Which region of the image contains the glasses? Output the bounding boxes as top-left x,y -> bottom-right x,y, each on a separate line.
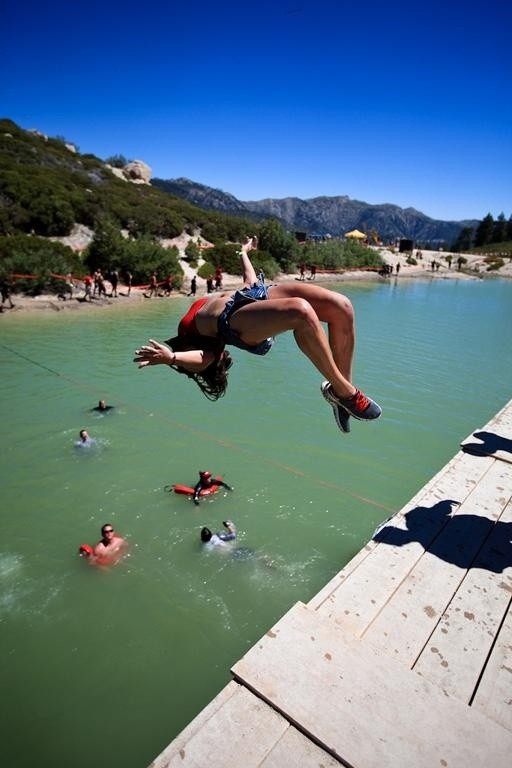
102,530 -> 114,533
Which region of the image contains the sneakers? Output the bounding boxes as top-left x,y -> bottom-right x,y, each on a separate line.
319,380 -> 382,433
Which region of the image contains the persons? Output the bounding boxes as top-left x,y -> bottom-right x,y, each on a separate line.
432,259 -> 435,273
310,261 -> 318,280
299,262 -> 308,280
55,268 -> 183,303
74,430 -> 97,450
0,277 -> 15,313
133,234 -> 383,434
193,471 -> 232,506
88,400 -> 113,416
416,252 -> 418,260
383,261 -> 401,276
198,521 -> 237,553
92,523 -> 126,559
206,263 -> 224,293
436,265 -> 439,271
259,267 -> 265,283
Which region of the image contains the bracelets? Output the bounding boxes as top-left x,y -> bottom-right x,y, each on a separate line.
170,354 -> 175,366
